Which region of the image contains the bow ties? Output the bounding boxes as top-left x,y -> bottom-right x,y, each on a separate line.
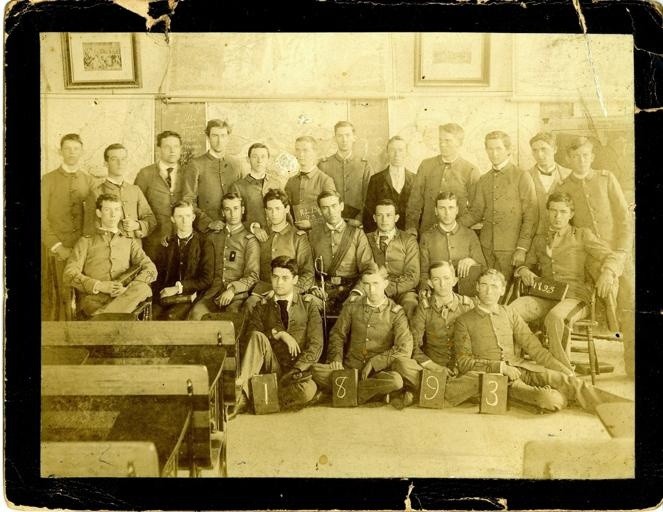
179,238 -> 188,242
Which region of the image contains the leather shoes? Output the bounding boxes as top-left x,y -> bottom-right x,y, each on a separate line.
305,390 -> 331,407
226,393 -> 248,419
402,388 -> 419,407
384,392 -> 404,410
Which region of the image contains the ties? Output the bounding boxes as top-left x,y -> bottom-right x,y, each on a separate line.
548,232 -> 558,249
166,168 -> 173,188
277,300 -> 289,329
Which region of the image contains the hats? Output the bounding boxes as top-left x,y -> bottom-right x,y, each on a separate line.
281,370 -> 313,386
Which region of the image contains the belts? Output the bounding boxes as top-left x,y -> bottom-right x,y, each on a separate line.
324,276 -> 349,286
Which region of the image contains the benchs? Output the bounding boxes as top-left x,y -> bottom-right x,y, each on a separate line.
41,320 -> 233,479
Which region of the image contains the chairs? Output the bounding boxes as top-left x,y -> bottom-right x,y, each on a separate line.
565,284 -> 599,381
51,255 -> 152,320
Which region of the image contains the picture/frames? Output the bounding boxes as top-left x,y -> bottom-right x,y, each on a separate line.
60,34 -> 144,91
413,31 -> 495,89
